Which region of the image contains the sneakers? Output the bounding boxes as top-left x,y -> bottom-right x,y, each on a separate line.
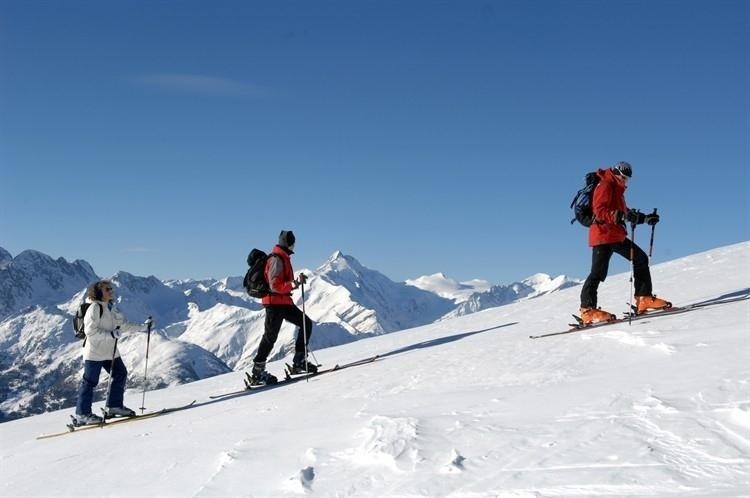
292,351 -> 317,372
579,307 -> 611,324
251,362 -> 277,385
103,406 -> 134,417
634,295 -> 667,313
75,412 -> 105,425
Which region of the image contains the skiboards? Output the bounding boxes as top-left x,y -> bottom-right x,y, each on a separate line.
36,399 -> 199,441
530,289 -> 750,338
209,356 -> 376,398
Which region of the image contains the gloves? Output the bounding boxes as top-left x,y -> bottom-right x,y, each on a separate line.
640,212 -> 658,225
293,273 -> 307,288
110,328 -> 122,339
615,209 -> 646,225
145,319 -> 156,331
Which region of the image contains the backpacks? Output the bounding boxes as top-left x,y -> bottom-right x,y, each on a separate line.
570,172 -> 601,228
238,247 -> 282,298
72,301 -> 103,345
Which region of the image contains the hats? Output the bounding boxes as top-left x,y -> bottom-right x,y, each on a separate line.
276,228 -> 294,249
614,161 -> 634,179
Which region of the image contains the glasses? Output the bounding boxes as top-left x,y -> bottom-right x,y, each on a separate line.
105,287 -> 113,292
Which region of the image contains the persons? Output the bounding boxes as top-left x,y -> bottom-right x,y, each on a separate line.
571,161 -> 672,323
243,230 -> 319,380
73,281 -> 136,423
